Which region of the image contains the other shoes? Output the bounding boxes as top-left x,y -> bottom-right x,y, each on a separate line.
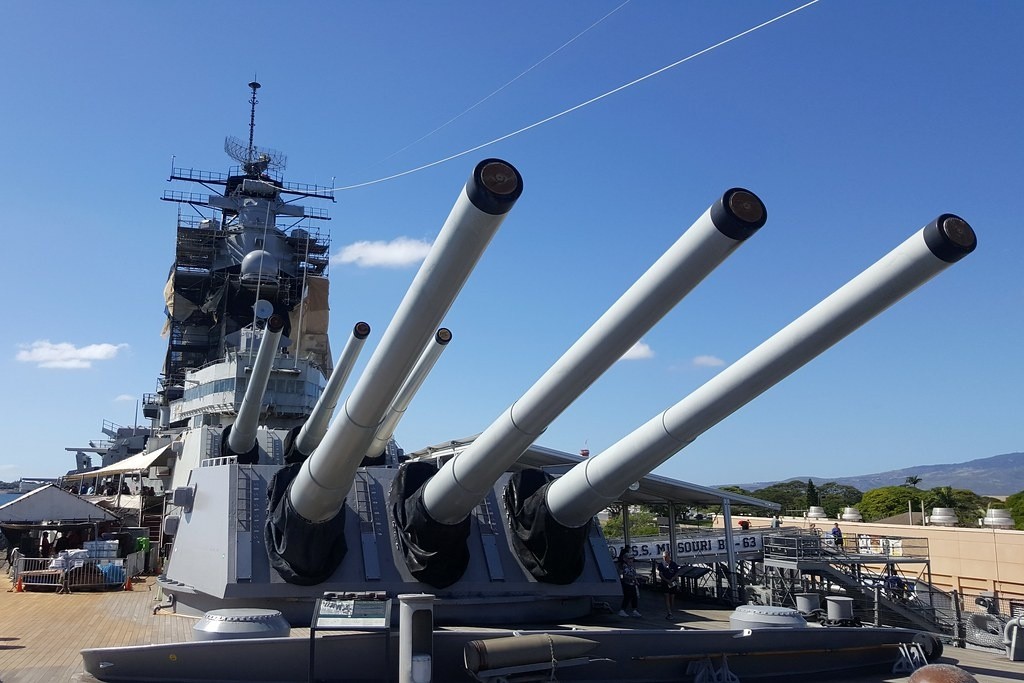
666,613 -> 675,620
618,610 -> 629,617
632,611 -> 642,617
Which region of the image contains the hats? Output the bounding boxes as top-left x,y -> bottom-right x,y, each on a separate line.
43,532 -> 49,535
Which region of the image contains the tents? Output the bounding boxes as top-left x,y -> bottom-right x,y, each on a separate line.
61,431 -> 183,526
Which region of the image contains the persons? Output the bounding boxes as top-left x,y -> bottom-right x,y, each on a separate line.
42,532 -> 54,559
55,531 -> 81,554
64,482 -> 131,495
